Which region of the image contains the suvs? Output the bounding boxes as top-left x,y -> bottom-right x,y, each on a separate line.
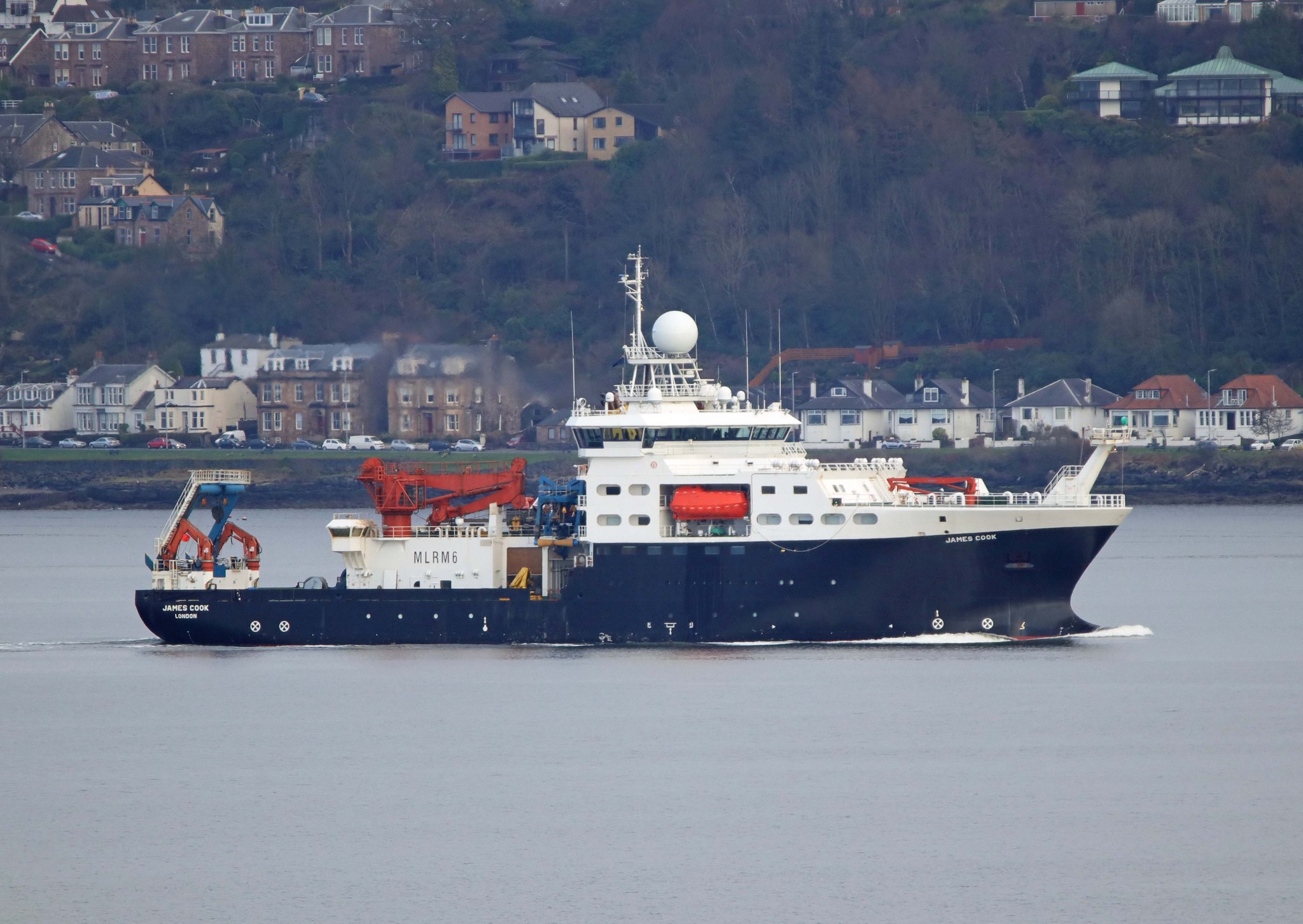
218,438 -> 270,450
390,440 -> 417,451
1280,439 -> 1302,451
429,439 -> 486,452
322,439 -> 350,451
881,438 -> 911,449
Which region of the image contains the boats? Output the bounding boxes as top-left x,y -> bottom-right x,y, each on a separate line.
133,244 -> 1135,645
670,486 -> 747,519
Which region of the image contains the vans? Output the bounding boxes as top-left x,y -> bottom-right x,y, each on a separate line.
349,435 -> 385,450
215,430 -> 246,446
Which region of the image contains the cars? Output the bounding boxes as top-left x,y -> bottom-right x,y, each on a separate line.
12,437 -> 52,448
290,440 -> 321,450
506,435 -> 522,447
89,437 -> 120,449
147,438 -> 186,450
1249,439 -> 1275,450
58,438 -> 86,449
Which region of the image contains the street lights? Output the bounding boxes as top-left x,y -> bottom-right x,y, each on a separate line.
791,372 -> 800,442
993,369 -> 1001,448
166,371 -> 173,449
20,370 -> 30,449
1209,369 -> 1217,441
